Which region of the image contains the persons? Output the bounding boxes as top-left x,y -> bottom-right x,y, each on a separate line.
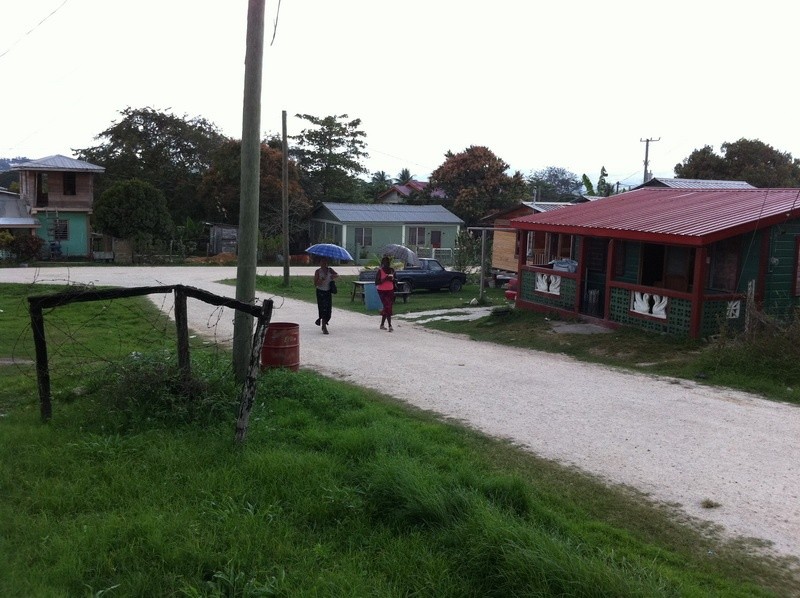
374,256 -> 398,332
314,258 -> 338,335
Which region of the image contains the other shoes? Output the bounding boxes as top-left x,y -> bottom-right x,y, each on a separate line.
315,319 -> 320,325
322,329 -> 329,334
388,326 -> 394,332
380,325 -> 387,330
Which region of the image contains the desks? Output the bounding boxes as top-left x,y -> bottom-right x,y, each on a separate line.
352,279 -> 412,306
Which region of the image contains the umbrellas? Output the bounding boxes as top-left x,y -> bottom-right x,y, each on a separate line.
380,244 -> 420,279
304,243 -> 354,280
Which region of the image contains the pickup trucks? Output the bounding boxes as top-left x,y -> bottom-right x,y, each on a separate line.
360,258 -> 467,293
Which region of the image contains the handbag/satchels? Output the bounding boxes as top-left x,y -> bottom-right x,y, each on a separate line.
329,280 -> 337,294
390,268 -> 399,290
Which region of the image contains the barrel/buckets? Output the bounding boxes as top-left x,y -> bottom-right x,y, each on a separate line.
261,323 -> 300,371
